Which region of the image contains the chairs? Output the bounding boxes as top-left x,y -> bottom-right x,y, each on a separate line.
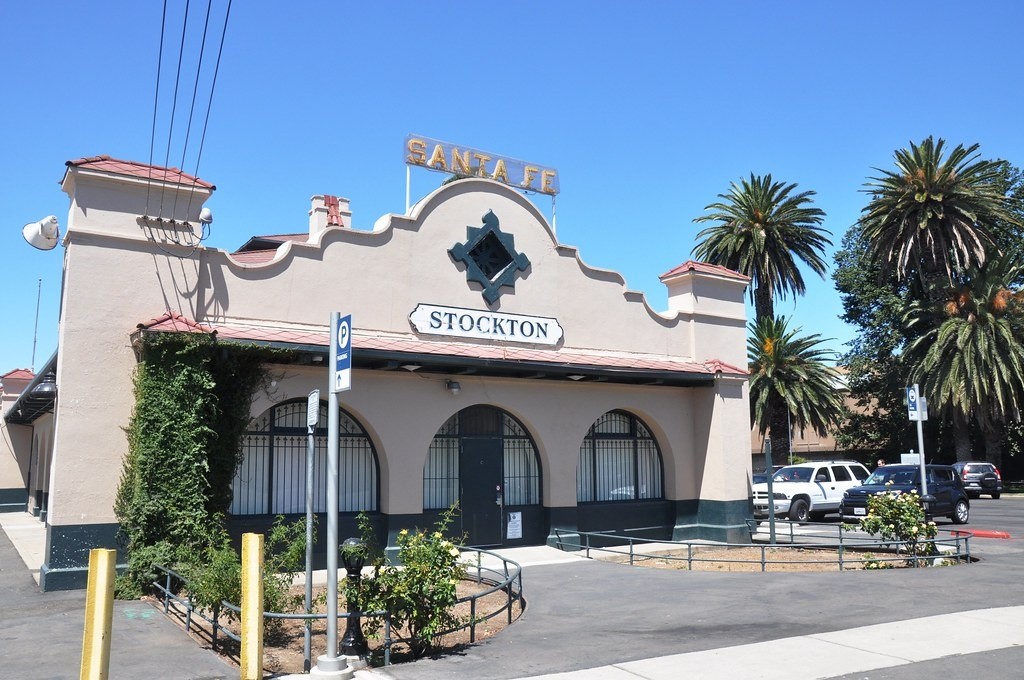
884,472 -> 901,485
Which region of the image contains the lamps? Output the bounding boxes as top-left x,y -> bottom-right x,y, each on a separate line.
310,354 -> 325,362
30,366 -> 60,397
23,215 -> 69,251
400,360 -> 423,372
446,381 -> 462,396
566,374 -> 590,382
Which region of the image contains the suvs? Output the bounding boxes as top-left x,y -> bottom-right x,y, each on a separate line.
753,461 -> 871,525
839,464 -> 970,524
951,462 -> 1002,500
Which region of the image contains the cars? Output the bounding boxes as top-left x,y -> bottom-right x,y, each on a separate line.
753,465 -> 787,484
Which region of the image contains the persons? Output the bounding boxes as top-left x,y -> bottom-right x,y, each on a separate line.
794,471 -> 800,478
872,459 -> 886,484
